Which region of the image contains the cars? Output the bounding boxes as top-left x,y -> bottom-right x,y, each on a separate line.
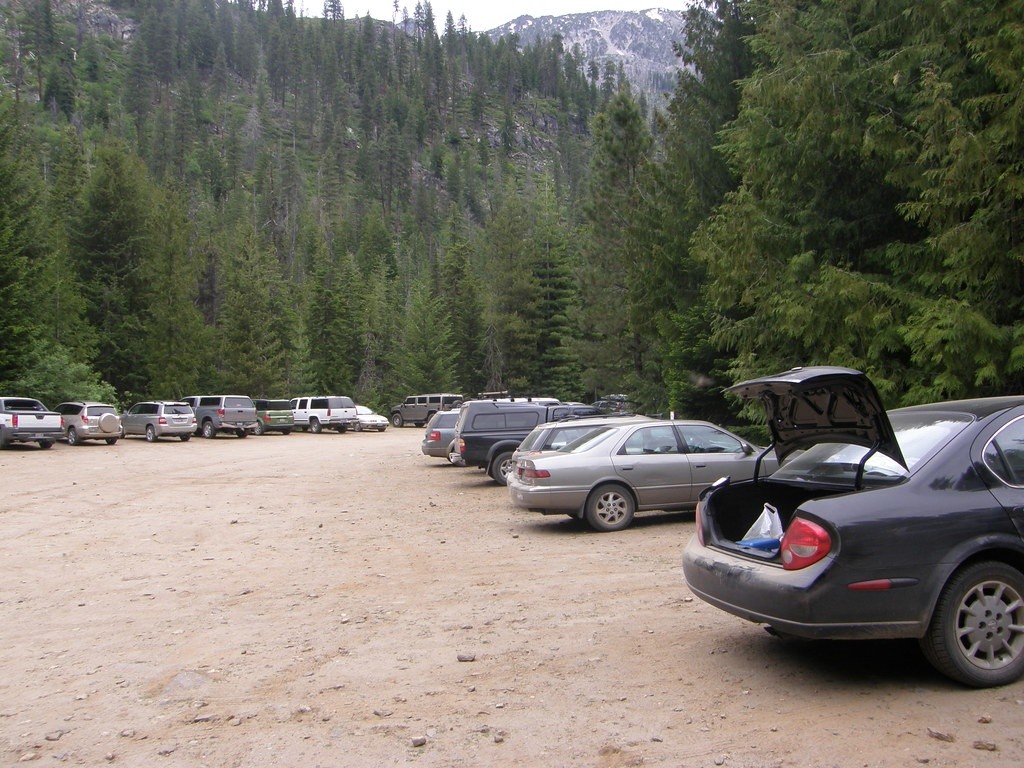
253,399 -> 295,435
681,364 -> 1024,687
355,405 -> 389,432
506,417 -> 808,530
421,395 -> 586,468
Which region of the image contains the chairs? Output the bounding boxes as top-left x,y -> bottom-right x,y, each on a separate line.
658,438 -> 674,453
644,438 -> 658,453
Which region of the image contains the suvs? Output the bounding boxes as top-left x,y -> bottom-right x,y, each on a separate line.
391,394 -> 464,428
54,401 -> 123,446
117,401 -> 197,442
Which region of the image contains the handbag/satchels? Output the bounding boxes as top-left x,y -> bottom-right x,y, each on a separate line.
742,503 -> 784,540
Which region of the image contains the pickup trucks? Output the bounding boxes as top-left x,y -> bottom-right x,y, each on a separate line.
455,401 -> 610,486
289,396 -> 358,434
179,394 -> 259,439
0,396 -> 65,450
